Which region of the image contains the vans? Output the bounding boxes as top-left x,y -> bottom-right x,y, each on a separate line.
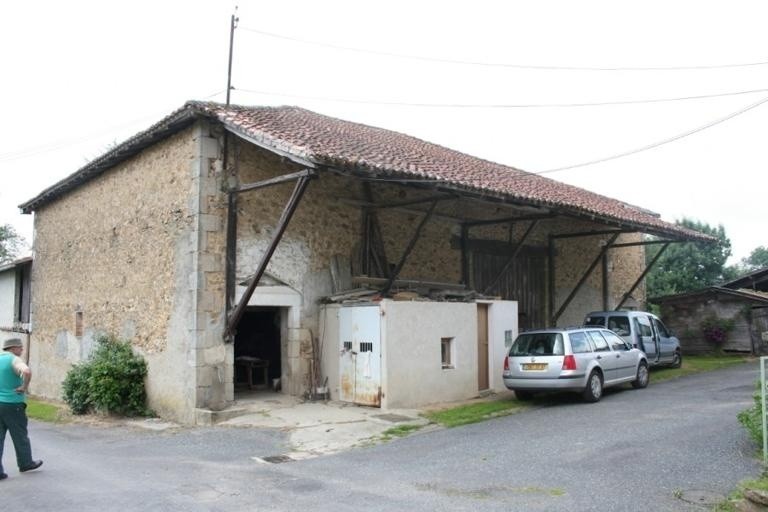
583,310 -> 682,370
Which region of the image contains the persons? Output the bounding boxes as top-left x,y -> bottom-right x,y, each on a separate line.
0,338 -> 44,479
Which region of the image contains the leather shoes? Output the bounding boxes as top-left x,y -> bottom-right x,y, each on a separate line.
0,474 -> 7,480
19,460 -> 42,472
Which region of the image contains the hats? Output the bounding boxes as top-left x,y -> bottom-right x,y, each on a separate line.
2,338 -> 22,351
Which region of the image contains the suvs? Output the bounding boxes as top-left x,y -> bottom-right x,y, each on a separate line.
503,323 -> 651,403
